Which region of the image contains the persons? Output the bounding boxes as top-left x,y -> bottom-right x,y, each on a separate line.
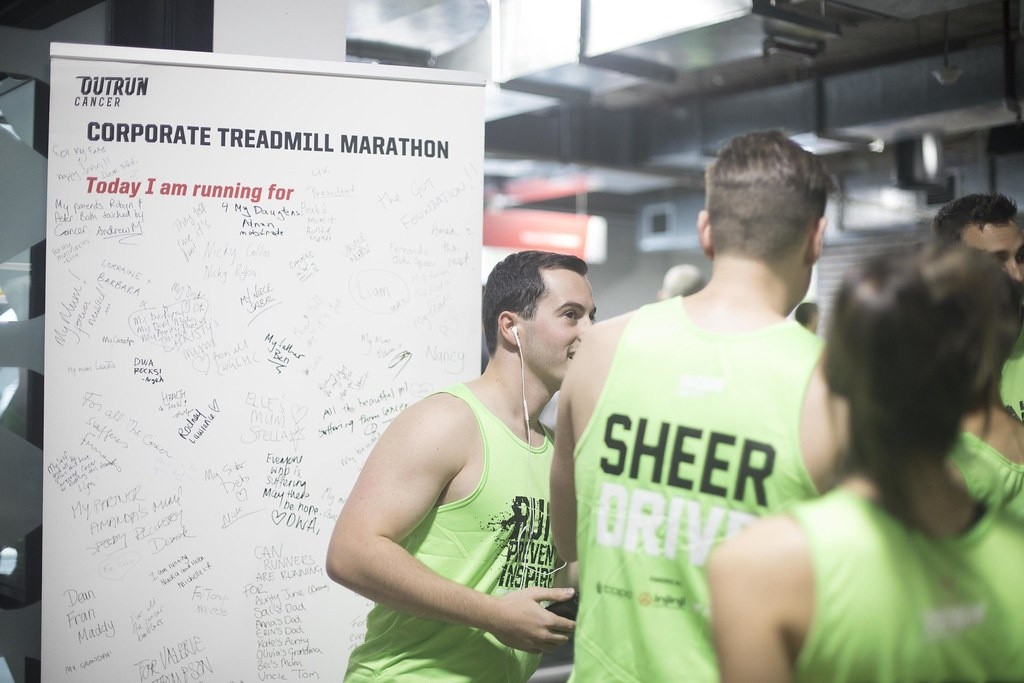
325,251 -> 597,682
795,302 -> 820,337
658,263 -> 704,302
546,128 -> 831,683
925,193 -> 1023,473
703,234 -> 1024,683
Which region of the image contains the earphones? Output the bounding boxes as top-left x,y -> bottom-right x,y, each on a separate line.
511,326 -> 521,348
